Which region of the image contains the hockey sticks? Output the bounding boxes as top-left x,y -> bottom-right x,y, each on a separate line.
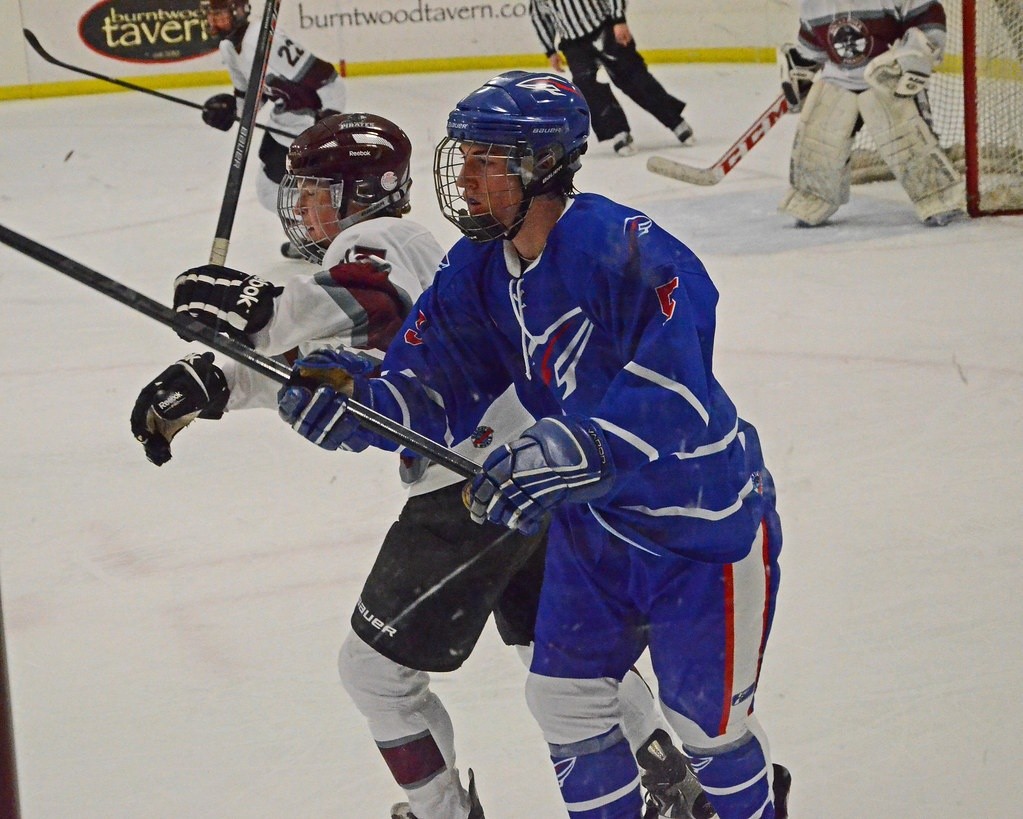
22,26 -> 298,139
0,225 -> 483,480
209,0 -> 282,266
646,93 -> 788,186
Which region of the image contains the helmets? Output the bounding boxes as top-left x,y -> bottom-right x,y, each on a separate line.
199,0 -> 252,43
277,113 -> 414,264
431,71 -> 592,245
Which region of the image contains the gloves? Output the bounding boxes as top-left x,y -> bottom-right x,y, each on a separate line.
273,349 -> 378,453
199,94 -> 237,132
170,264 -> 286,348
130,352 -> 230,467
466,415 -> 604,533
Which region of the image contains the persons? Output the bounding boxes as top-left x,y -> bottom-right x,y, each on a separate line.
529,0 -> 696,157
776,0 -> 967,227
199,0 -> 345,185
277,70 -> 791,819
129,114 -> 714,819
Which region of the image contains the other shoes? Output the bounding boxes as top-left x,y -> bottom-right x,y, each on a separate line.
647,759 -> 717,819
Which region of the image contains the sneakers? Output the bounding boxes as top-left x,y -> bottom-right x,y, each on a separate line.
612,133 -> 641,157
671,120 -> 697,147
